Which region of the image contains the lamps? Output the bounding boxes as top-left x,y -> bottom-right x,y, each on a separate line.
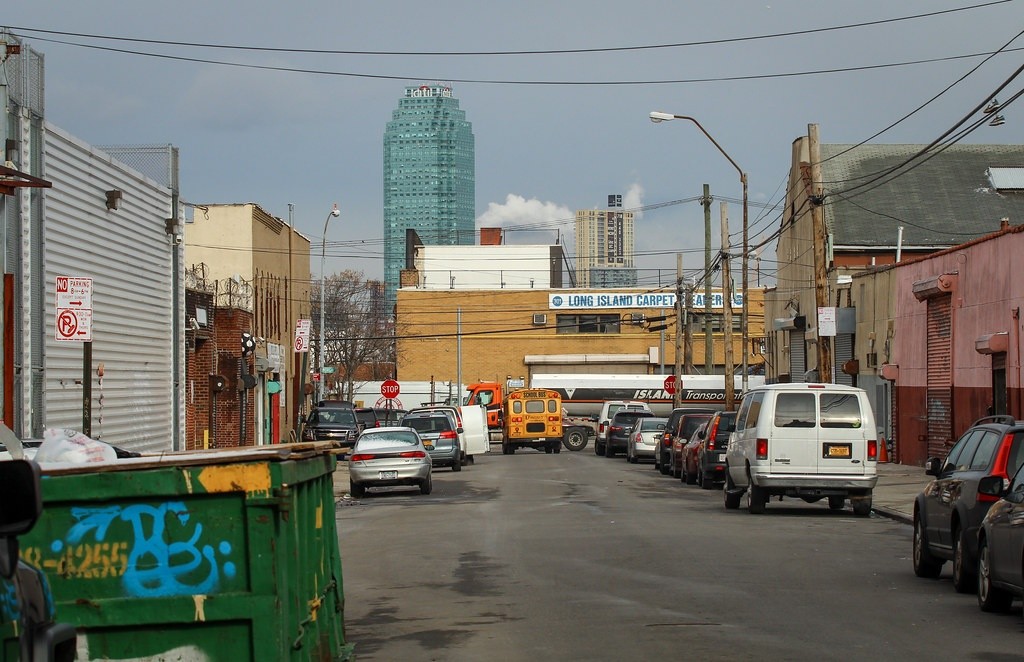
164,217 -> 179,235
106,188 -> 123,211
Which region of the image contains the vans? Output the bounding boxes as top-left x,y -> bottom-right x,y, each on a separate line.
718,383 -> 878,514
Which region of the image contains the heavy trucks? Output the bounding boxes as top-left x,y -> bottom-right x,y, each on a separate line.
463,383 -> 599,451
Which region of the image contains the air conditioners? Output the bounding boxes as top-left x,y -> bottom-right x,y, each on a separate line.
631,313 -> 645,324
533,314 -> 547,325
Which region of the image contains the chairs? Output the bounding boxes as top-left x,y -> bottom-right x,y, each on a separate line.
314,415 -> 324,422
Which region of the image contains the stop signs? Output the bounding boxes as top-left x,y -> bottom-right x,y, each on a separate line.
381,380 -> 400,399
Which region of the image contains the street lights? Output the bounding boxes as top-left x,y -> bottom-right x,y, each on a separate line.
649,111 -> 748,396
319,210 -> 341,401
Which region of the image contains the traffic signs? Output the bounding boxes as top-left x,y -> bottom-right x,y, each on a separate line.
54,276 -> 93,308
295,318 -> 310,336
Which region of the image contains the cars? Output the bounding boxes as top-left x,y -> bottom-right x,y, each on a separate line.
300,407 -> 359,461
599,394 -> 738,489
356,408 -> 468,472
347,427 -> 432,497
913,415 -> 1024,594
976,463 -> 1024,612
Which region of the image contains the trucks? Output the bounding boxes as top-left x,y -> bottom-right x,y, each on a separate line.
498,388 -> 563,454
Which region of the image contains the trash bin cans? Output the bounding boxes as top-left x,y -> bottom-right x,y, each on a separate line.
16,451 -> 356,662
